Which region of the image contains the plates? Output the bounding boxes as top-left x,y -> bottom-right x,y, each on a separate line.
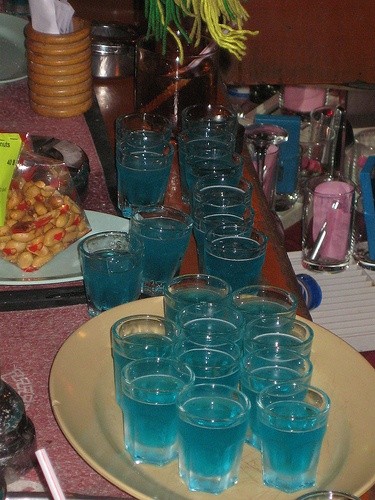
46,294 -> 375,500
0,209 -> 130,286
0,12 -> 29,84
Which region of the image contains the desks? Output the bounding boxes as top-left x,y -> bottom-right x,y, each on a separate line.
0,0 -> 313,500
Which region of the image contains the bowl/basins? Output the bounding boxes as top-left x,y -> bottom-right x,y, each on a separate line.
93,21 -> 140,80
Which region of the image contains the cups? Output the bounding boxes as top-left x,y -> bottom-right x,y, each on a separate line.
128,203 -> 194,297
176,127 -> 236,205
131,30 -> 222,141
244,84 -> 375,272
203,223 -> 268,290
114,112 -> 173,171
181,103 -> 237,133
110,272 -> 330,494
24,16 -> 93,119
190,172 -> 255,215
192,196 -> 255,274
116,129 -> 175,218
78,231 -> 145,317
184,147 -> 243,190
